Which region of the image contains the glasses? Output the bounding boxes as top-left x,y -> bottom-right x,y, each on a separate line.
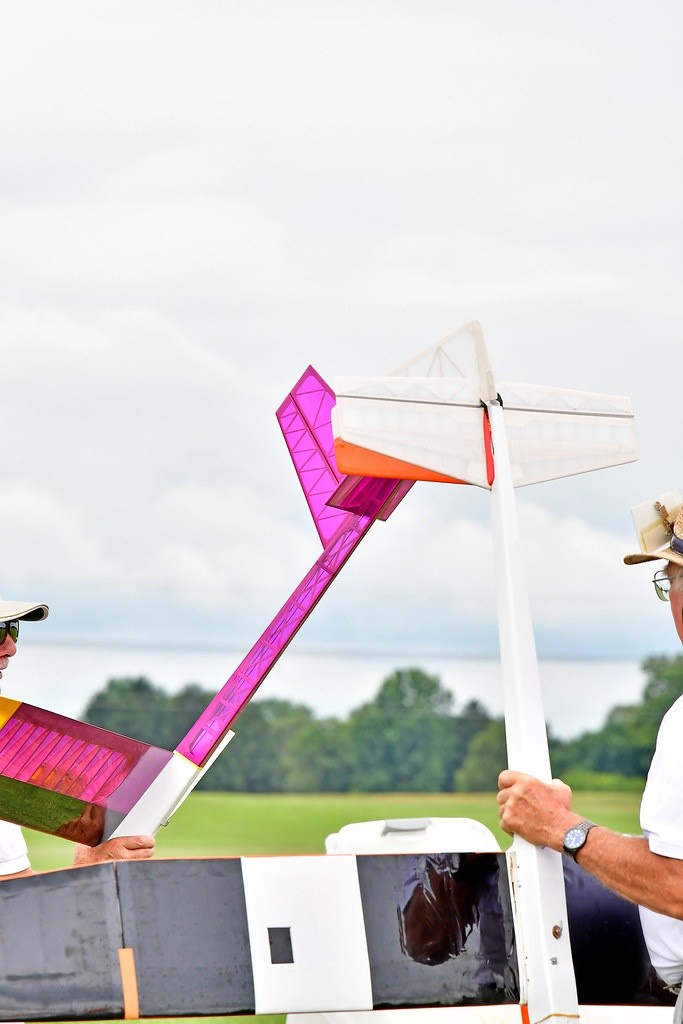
652,570 -> 683,601
0,619 -> 19,645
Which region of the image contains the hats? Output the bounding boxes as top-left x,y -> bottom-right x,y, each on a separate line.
624,503 -> 683,567
0,595 -> 49,623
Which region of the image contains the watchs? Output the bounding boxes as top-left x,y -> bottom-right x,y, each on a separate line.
562,820 -> 598,864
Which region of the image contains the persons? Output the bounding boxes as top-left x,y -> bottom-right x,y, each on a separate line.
497,489 -> 683,1024
399,853 -> 499,1006
0,596 -> 155,882
